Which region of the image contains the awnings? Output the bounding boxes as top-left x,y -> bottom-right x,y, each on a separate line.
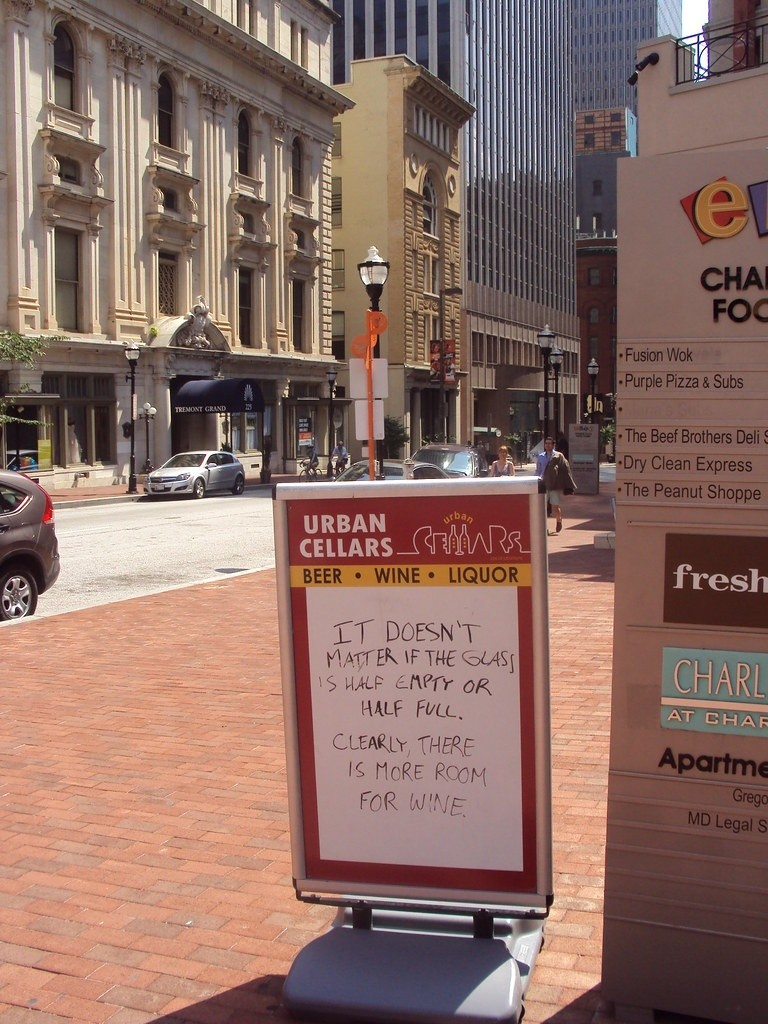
173,377 -> 265,414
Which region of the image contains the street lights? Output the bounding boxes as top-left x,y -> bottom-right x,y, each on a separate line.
586,358 -> 601,426
141,403 -> 157,474
535,324 -> 564,461
124,342 -> 140,495
359,245 -> 388,476
327,364 -> 338,480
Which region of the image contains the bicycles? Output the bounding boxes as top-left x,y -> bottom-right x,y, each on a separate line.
297,460 -> 326,481
331,459 -> 350,475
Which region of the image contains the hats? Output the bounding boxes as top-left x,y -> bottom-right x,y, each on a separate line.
339,441 -> 343,446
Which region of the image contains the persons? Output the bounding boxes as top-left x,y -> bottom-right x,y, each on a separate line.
302,442 -> 319,481
535,437 -> 577,533
329,440 -> 348,476
488,445 -> 515,476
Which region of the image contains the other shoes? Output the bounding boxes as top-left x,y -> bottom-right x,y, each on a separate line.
556,518 -> 562,532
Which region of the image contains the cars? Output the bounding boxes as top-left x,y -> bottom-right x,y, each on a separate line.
329,459 -> 451,481
0,469 -> 60,622
408,444 -> 491,478
142,451 -> 246,501
